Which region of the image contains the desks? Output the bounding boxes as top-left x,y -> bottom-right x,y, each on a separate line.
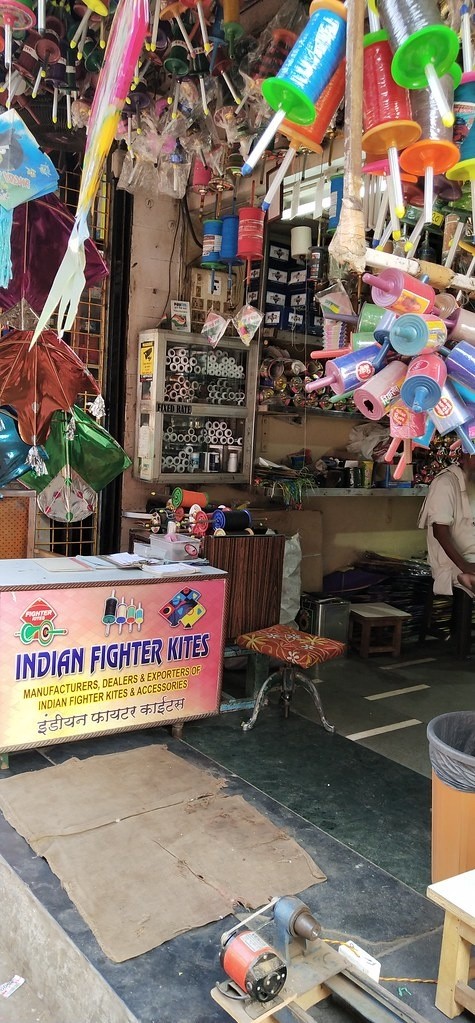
0,555 -> 230,770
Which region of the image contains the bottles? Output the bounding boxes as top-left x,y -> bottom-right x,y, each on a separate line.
227,453 -> 238,472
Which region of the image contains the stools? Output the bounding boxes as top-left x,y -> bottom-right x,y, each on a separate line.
350,601 -> 412,658
427,871 -> 474,1019
236,624 -> 344,732
417,578 -> 475,662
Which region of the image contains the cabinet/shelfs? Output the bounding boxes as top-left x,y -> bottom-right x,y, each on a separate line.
191,140 -> 475,499
132,329 -> 258,485
130,528 -> 286,712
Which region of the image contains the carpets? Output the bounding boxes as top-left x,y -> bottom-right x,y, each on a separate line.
0,745 -> 326,964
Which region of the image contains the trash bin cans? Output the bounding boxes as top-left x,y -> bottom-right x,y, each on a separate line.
426,710 -> 475,884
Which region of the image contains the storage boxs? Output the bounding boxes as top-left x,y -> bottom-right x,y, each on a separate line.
245,241 -> 328,336
373,462 -> 415,489
134,533 -> 200,560
339,460 -> 374,489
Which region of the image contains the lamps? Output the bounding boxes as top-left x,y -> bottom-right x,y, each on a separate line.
290,225 -> 313,264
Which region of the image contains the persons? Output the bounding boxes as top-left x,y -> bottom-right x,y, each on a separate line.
417,444 -> 475,669
